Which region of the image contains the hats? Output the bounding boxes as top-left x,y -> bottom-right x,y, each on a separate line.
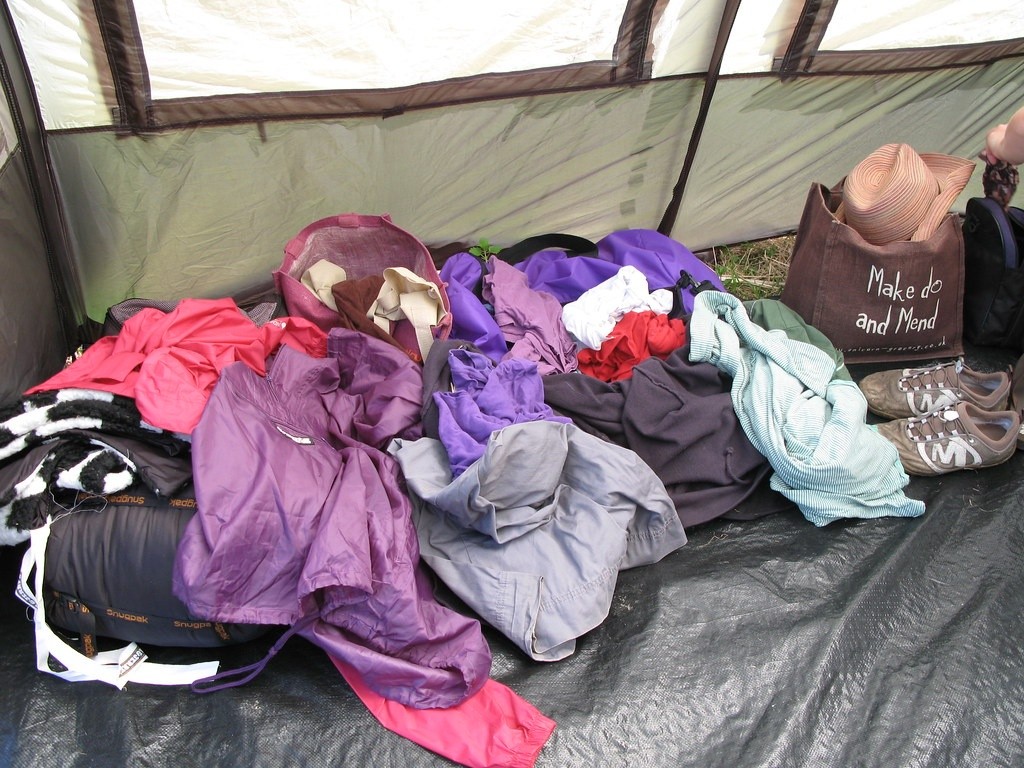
834,142 -> 976,244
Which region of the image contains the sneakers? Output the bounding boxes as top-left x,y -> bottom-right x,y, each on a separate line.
857,357 -> 1012,419
873,400 -> 1019,477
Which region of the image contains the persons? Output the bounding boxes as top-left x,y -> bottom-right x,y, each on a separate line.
987,106 -> 1024,451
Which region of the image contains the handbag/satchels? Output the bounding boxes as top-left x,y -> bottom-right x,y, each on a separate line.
958,194 -> 1024,352
272,212 -> 454,363
780,173 -> 964,363
